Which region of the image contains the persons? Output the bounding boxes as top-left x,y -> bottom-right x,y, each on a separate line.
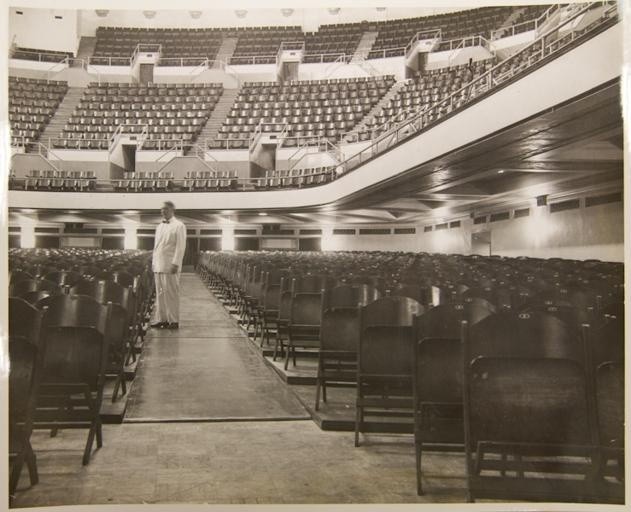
150,200 -> 187,330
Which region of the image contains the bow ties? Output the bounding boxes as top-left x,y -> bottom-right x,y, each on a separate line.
161,220 -> 171,225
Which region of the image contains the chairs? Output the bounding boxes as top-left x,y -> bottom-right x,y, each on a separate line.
583,324 -> 625,507
459,317 -> 606,505
196,251 -> 625,448
411,312 -> 524,496
8,245 -> 157,497
22,165 -> 337,193
10,5 -> 557,149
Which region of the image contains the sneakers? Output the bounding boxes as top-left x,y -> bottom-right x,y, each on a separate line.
150,321 -> 179,329
